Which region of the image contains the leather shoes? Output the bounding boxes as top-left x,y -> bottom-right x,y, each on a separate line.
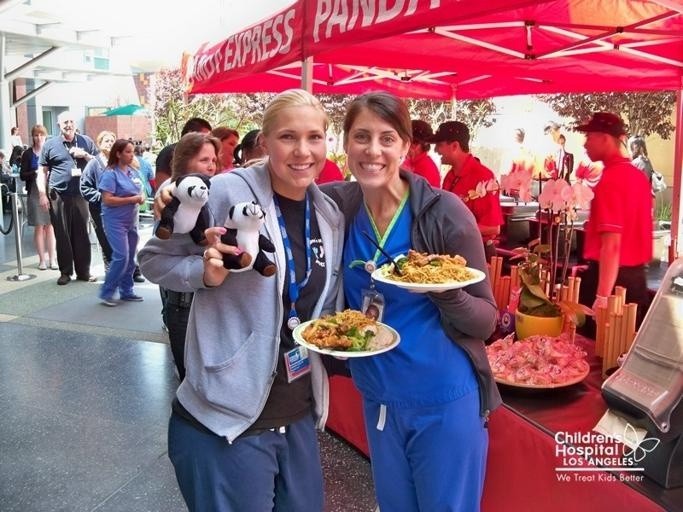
57,273 -> 70,285
38,263 -> 47,270
49,262 -> 59,270
77,274 -> 97,282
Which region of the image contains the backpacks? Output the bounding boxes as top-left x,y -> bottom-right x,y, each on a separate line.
646,157 -> 666,193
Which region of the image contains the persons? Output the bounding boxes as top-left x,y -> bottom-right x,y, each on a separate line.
19,124 -> 58,270
78,131 -> 144,283
153,92 -> 501,512
159,132 -> 226,384
134,86 -> 343,512
34,111 -> 98,285
157,118 -> 668,332
570,111 -> 653,342
0,127 -> 155,224
97,139 -> 143,307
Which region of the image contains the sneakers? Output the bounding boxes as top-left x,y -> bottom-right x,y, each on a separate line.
98,296 -> 118,306
120,296 -> 144,302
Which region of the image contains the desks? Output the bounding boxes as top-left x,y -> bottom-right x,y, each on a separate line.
327,371 -> 681,511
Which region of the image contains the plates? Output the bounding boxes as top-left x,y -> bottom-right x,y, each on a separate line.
370,264 -> 483,298
288,317 -> 401,360
491,359 -> 592,390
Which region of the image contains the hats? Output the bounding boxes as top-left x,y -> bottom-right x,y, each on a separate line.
426,121 -> 469,143
411,119 -> 432,139
573,111 -> 625,134
